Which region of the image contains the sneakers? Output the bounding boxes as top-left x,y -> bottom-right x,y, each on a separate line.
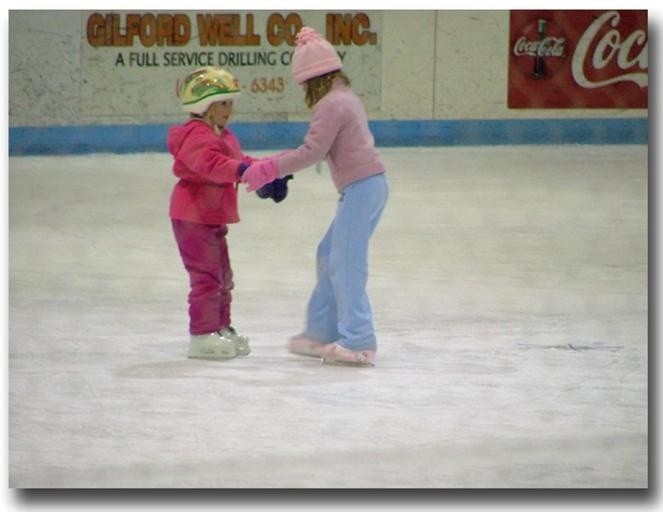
218,327 -> 249,351
189,331 -> 235,355
321,341 -> 375,362
289,334 -> 326,356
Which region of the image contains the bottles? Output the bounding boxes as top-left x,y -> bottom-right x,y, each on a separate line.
532,18 -> 548,76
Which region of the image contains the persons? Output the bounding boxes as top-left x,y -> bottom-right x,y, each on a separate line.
168,67 -> 291,361
241,25 -> 389,370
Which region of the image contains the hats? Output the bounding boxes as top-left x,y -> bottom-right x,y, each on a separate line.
291,26 -> 341,85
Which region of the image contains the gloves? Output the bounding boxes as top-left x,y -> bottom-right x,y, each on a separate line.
256,174 -> 294,203
240,158 -> 278,192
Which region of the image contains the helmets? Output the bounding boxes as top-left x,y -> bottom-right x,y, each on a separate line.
176,65 -> 241,117
